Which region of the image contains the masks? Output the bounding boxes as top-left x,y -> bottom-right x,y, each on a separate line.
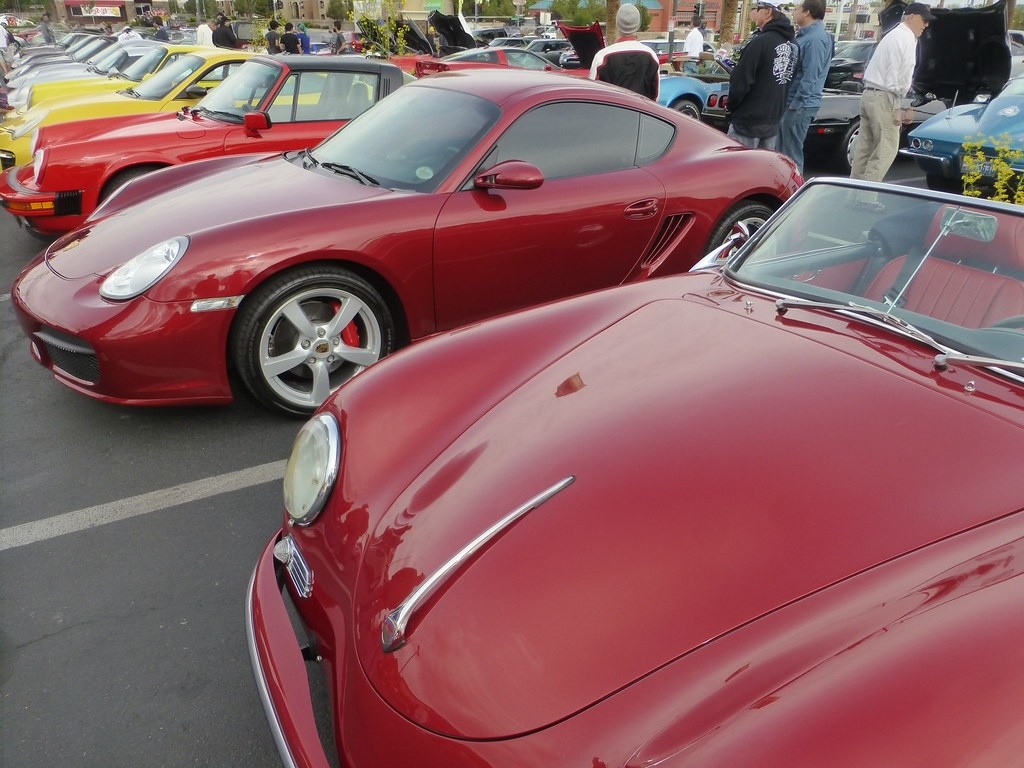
333,28 -> 336,32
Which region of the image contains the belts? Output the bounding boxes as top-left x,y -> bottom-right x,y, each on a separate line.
863,86 -> 881,91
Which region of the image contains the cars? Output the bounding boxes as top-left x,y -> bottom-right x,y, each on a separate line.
242,171 -> 1024,767
0,10 -> 1023,246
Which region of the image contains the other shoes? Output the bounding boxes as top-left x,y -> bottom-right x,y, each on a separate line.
853,201 -> 887,214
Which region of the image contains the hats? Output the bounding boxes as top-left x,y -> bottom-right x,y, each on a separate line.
219,15 -> 231,23
752,0 -> 782,12
615,3 -> 642,34
902,3 -> 938,20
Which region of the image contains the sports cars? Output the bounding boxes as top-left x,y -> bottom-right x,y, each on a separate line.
9,62 -> 804,422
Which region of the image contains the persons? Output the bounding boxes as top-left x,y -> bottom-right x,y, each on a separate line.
846,3 -> 937,183
265,20 -> 280,54
332,21 -> 346,55
588,3 -> 660,102
152,16 -> 168,40
682,16 -> 704,74
196,12 -> 237,50
775,0 -> 833,179
280,22 -> 304,56
727,0 -> 800,150
295,22 -> 311,56
42,13 -> 56,44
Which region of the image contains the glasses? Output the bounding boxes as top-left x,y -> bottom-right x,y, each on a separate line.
757,5 -> 769,11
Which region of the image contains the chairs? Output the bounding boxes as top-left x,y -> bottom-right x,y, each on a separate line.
866,202 -> 1024,331
